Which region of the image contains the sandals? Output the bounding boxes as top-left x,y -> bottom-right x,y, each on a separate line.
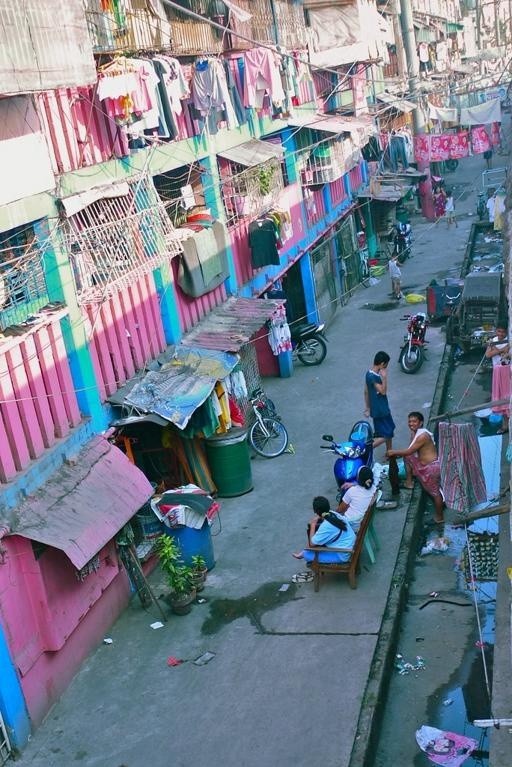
292,571 -> 315,579
292,576 -> 313,583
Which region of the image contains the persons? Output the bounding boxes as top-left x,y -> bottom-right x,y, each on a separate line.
387,252 -> 405,302
361,350 -> 395,452
485,152 -> 496,170
385,412 -> 447,524
484,323 -> 511,365
290,495 -> 358,566
336,465 -> 375,525
443,190 -> 461,230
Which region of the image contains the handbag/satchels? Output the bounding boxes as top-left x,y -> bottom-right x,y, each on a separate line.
307,519 -> 326,547
336,487 -> 348,505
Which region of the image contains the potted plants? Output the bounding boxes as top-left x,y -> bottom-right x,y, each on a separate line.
152,533 -> 208,616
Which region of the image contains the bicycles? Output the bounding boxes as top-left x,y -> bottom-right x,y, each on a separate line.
249,389 -> 289,458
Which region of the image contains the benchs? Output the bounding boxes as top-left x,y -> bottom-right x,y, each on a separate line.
303,489 -> 379,593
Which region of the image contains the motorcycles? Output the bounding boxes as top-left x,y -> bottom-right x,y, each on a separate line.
398,313 -> 429,374
291,323 -> 327,366
319,421 -> 372,488
391,220 -> 411,263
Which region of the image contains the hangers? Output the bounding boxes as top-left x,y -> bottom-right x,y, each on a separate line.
99,49 -> 160,72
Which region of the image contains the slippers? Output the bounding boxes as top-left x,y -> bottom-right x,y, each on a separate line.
399,482 -> 414,490
496,427 -> 509,434
424,518 -> 444,525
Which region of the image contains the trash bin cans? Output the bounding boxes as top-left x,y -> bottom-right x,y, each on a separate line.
202,427 -> 255,497
